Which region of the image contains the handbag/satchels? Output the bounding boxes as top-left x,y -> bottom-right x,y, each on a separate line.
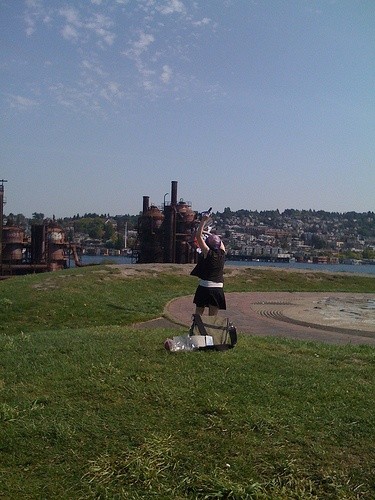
188,313 -> 237,349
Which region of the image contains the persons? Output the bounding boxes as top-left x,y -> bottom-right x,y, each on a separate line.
190,212 -> 227,316
